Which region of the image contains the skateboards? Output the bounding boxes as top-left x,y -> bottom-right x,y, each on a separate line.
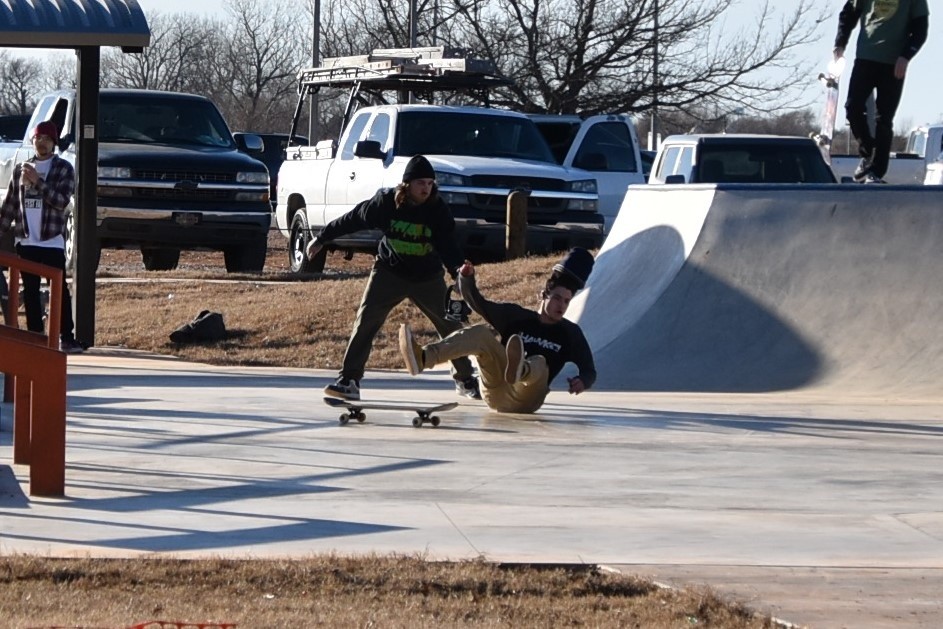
322,397 -> 460,428
808,56 -> 848,166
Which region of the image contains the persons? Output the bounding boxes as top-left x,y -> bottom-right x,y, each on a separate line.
0,121 -> 83,353
399,259 -> 597,414
834,0 -> 929,184
306,154 -> 482,401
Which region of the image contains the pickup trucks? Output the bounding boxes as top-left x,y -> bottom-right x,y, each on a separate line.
830,122 -> 943,185
0,87 -> 274,273
275,48 -> 658,281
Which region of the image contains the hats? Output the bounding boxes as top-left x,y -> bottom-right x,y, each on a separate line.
35,121 -> 56,141
403,155 -> 435,183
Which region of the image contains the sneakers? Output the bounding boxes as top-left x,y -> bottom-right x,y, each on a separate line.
504,335 -> 525,384
325,375 -> 360,400
853,158 -> 871,181
62,339 -> 82,353
453,377 -> 482,400
398,324 -> 423,376
864,173 -> 882,184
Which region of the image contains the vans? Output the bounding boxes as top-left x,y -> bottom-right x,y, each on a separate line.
231,132 -> 308,208
649,133 -> 837,185
